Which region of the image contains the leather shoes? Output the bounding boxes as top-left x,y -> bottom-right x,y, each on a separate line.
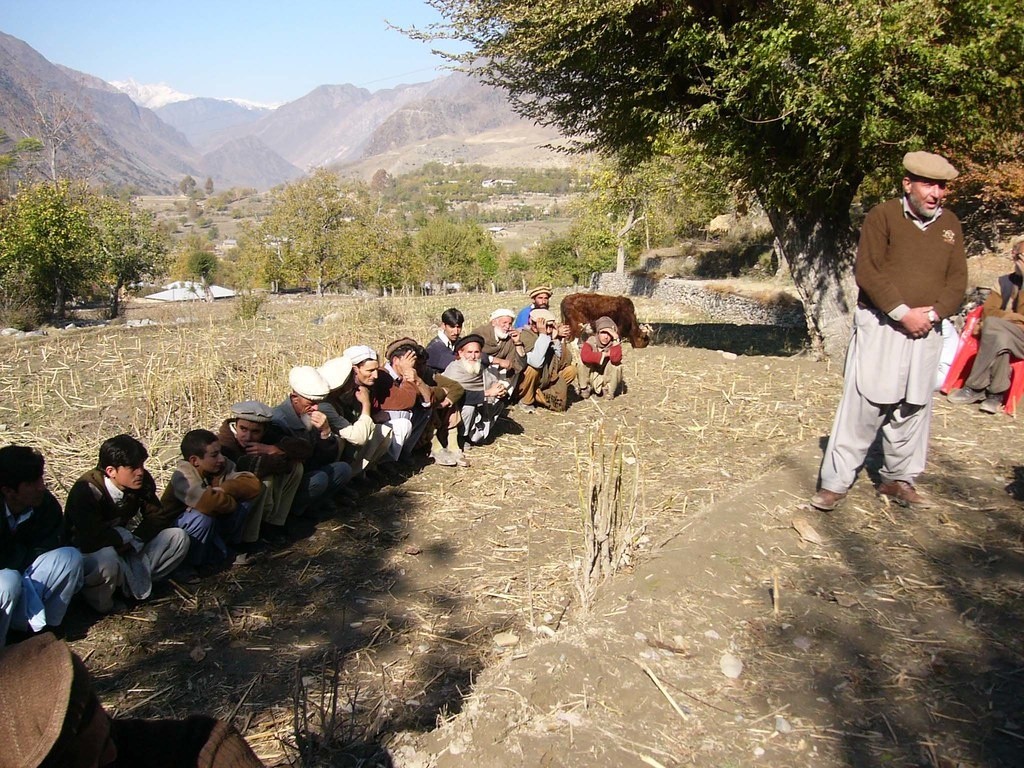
877,480 -> 931,508
810,489 -> 847,510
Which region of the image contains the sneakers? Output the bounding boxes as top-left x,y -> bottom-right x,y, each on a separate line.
447,449 -> 470,466
980,394 -> 1004,414
948,386 -> 986,405
429,448 -> 456,466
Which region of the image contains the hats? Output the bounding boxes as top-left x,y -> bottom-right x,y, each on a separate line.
232,400 -> 274,424
384,337 -> 418,360
0,630 -> 74,768
596,316 -> 617,333
489,309 -> 516,319
530,309 -> 555,325
454,334 -> 485,352
903,151 -> 960,181
290,366 -> 330,401
343,345 -> 377,364
318,356 -> 353,392
528,286 -> 553,299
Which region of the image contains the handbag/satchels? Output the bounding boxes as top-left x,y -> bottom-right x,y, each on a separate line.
535,375 -> 567,412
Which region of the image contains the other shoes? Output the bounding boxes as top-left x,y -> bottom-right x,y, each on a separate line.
579,386 -> 591,399
111,449 -> 426,613
518,400 -> 536,414
602,392 -> 615,400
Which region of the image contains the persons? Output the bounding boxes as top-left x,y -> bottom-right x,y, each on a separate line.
472,308 -> 527,400
218,399 -> 304,555
162,429 -> 261,584
514,286 -> 553,330
947,234 -> 1024,413
809,151 -> 968,509
317,337 -> 470,497
0,446 -> 84,648
441,334 -> 508,443
520,309 -> 577,410
426,307 -> 513,372
64,434 -> 189,613
265,365 -> 351,522
578,316 -> 623,399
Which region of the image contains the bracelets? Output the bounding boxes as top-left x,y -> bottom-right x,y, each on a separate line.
929,310 -> 938,322
514,342 -> 524,346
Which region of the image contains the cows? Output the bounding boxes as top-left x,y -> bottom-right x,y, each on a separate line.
560,293 -> 650,348
